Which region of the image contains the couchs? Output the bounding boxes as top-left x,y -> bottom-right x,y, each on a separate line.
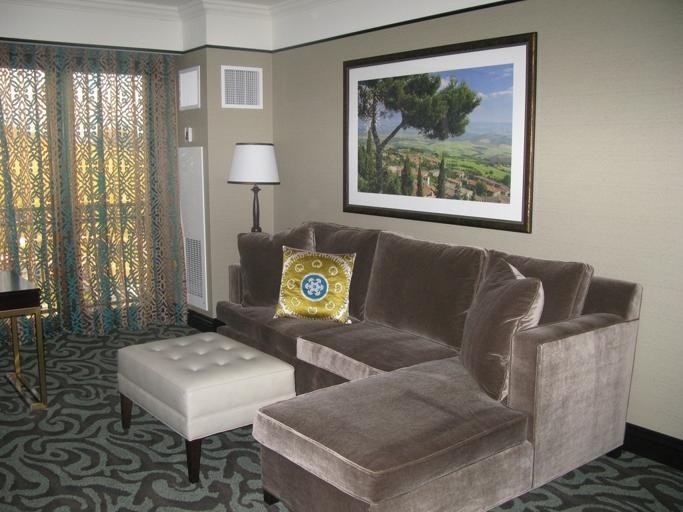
217,222 -> 643,512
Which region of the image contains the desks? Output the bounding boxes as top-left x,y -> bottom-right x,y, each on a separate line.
0,271 -> 48,412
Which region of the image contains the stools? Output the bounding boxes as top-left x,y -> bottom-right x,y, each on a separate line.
116,331 -> 297,484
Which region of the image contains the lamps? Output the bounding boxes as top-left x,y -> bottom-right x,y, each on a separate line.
227,143 -> 281,234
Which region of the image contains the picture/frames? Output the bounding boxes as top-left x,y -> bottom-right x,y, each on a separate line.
342,31 -> 537,235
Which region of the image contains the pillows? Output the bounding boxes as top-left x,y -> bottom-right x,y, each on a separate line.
459,259 -> 544,404
236,227 -> 356,325
